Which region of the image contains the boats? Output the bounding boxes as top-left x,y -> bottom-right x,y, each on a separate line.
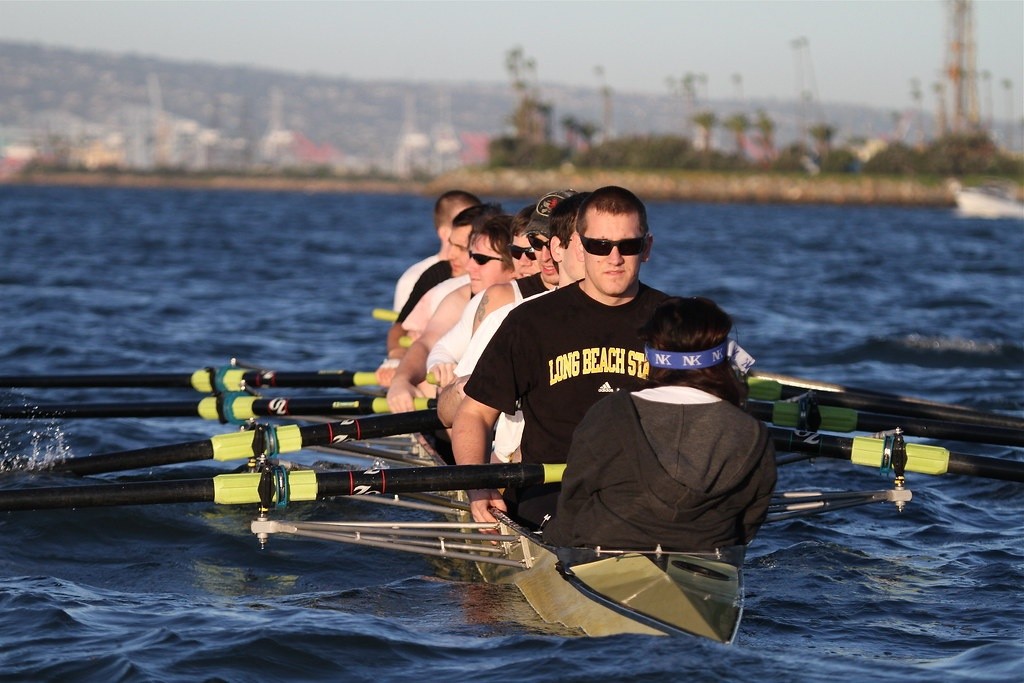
416,438 -> 751,651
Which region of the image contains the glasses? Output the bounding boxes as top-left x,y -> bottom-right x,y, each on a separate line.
508,243 -> 537,261
468,250 -> 503,265
527,234 -> 552,252
581,233 -> 647,256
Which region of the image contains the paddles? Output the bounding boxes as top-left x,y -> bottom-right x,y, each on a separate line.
746,375 -> 1022,447
2,394 -> 437,424
766,426 -> 1022,485
45,409 -> 438,476
0,462 -> 572,517
1,360 -> 378,395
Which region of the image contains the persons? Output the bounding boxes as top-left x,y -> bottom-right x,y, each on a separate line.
452,186 -> 671,544
473,189 -> 577,343
377,274 -> 471,387
383,214 -> 514,414
387,204 -> 485,361
543,297 -> 776,553
425,204 -> 536,389
392,190 -> 481,314
437,192 -> 592,463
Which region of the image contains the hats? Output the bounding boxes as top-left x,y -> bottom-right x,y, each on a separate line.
524,188 -> 578,234
